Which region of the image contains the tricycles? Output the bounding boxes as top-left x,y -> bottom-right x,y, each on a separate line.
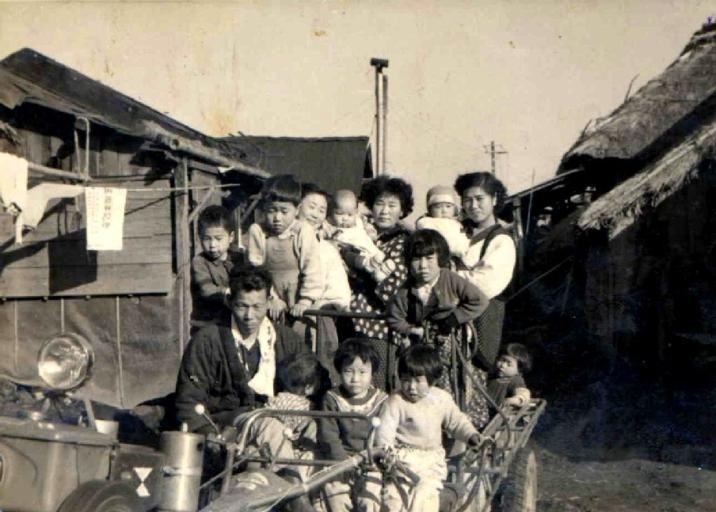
2,304 -> 548,512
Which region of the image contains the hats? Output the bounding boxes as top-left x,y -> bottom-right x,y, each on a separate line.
426,186 -> 463,216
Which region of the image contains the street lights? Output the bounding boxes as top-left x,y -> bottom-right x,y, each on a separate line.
370,56 -> 388,175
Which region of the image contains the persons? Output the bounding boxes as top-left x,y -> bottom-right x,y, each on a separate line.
379,343 -> 495,511
168,265 -> 333,481
185,171 -> 529,408
261,354 -> 322,480
315,336 -> 394,512
